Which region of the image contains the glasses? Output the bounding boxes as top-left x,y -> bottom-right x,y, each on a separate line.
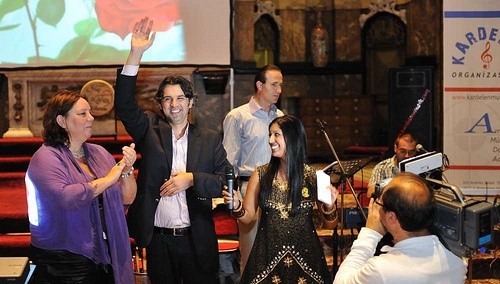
375,198 -> 392,212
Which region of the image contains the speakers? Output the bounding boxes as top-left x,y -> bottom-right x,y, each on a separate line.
387,65 -> 434,159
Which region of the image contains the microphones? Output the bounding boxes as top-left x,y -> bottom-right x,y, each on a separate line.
226,166 -> 235,215
415,144 -> 430,154
315,117 -> 328,131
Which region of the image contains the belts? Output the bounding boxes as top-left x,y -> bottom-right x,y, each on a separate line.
154,226 -> 191,236
239,176 -> 250,181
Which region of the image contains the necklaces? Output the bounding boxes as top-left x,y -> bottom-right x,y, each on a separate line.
67,145 -> 85,159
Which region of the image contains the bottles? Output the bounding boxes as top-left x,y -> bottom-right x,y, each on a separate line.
310,5 -> 329,68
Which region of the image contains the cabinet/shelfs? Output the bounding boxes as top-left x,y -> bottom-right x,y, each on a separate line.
300,95 -> 365,158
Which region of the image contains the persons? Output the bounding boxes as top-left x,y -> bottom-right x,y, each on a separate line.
222,114 -> 339,284
221,64 -> 286,278
366,132 -> 418,201
113,14 -> 228,284
332,171 -> 468,284
16,89 -> 139,284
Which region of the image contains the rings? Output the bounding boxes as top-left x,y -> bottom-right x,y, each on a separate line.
118,162 -> 120,166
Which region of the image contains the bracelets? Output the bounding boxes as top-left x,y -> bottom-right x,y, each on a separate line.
120,167 -> 134,178
232,200 -> 247,220
